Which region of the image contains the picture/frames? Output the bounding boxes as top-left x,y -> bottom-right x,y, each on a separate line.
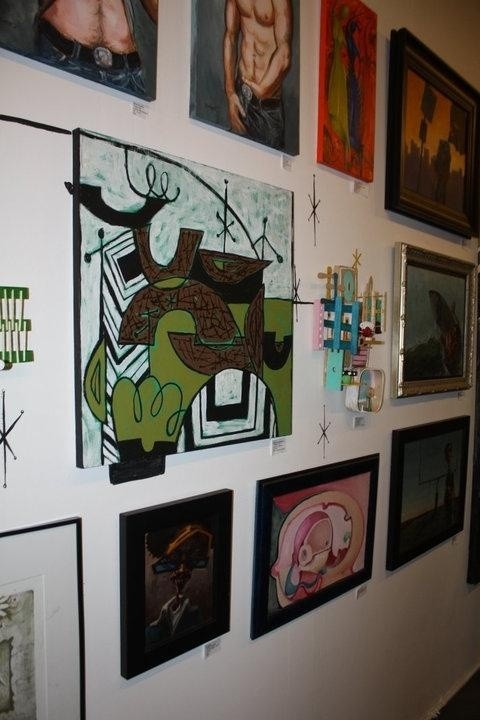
381,240 -> 477,399
386,28 -> 480,240
1,517 -> 89,718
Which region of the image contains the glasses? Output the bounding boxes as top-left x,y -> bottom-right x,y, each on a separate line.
150,552 -> 209,575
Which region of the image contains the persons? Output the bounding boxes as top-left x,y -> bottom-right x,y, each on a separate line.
223,1 -> 291,150
345,19 -> 365,156
38,0 -> 158,97
327,0 -> 351,152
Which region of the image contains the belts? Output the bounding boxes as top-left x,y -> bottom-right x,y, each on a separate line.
34,17 -> 142,73
235,71 -> 281,110
